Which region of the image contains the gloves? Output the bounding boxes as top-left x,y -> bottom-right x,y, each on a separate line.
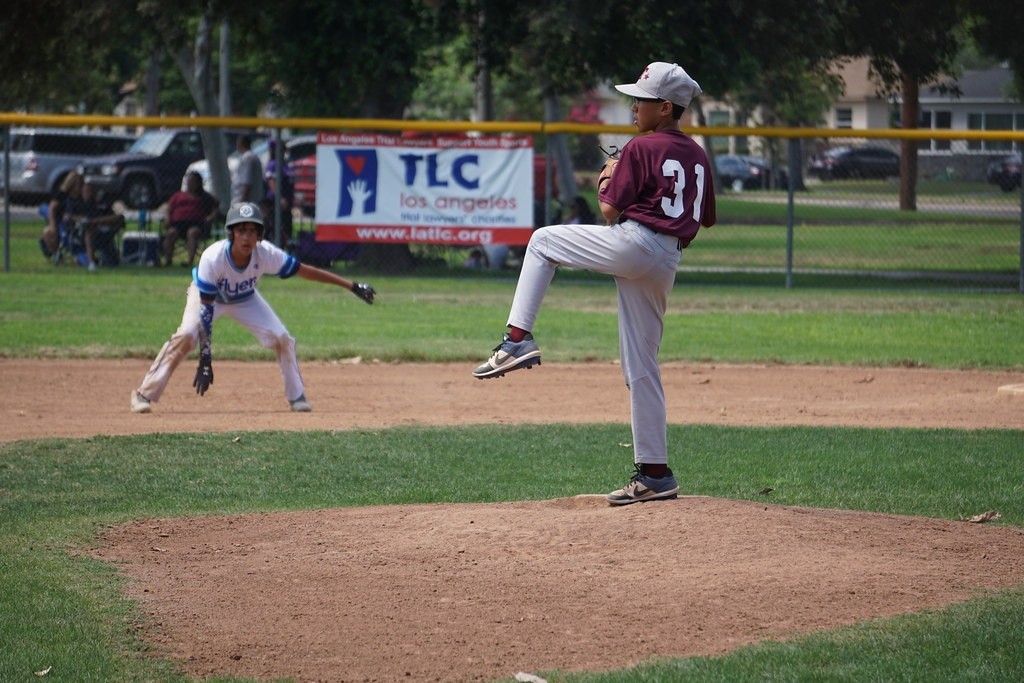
191,360 -> 214,396
351,282 -> 376,305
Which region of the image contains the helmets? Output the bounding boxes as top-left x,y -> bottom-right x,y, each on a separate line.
225,202 -> 264,237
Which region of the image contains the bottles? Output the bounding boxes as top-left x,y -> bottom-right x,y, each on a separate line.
158,217 -> 166,238
138,206 -> 152,231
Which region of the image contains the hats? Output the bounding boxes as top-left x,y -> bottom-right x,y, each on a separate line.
615,62 -> 702,109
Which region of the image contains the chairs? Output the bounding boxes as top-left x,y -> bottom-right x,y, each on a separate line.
39,196 -> 221,269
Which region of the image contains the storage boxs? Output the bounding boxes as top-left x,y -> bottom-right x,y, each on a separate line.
124,231 -> 160,266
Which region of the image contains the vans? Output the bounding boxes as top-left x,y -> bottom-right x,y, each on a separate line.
0,127 -> 138,200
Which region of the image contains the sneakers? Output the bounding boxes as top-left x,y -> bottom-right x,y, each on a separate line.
472,332 -> 541,381
605,462 -> 679,503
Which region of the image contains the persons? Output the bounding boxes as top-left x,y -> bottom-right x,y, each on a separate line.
473,62 -> 716,505
43,172 -> 124,271
162,133 -> 295,268
806,156 -> 840,182
130,202 -> 376,413
564,197 -> 594,224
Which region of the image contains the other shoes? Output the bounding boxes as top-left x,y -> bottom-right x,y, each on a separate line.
130,390 -> 151,413
87,261 -> 96,272
289,396 -> 311,412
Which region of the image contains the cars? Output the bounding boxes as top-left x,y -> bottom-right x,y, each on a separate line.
987,154 -> 1022,191
808,144 -> 901,182
715,155 -> 786,194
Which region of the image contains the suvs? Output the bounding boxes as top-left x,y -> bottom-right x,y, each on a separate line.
181,133 -> 315,209
78,129 -> 276,207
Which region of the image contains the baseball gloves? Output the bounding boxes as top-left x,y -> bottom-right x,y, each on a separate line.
596,149 -> 622,188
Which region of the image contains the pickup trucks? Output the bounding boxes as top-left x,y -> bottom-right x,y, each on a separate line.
287,153 -> 559,215
